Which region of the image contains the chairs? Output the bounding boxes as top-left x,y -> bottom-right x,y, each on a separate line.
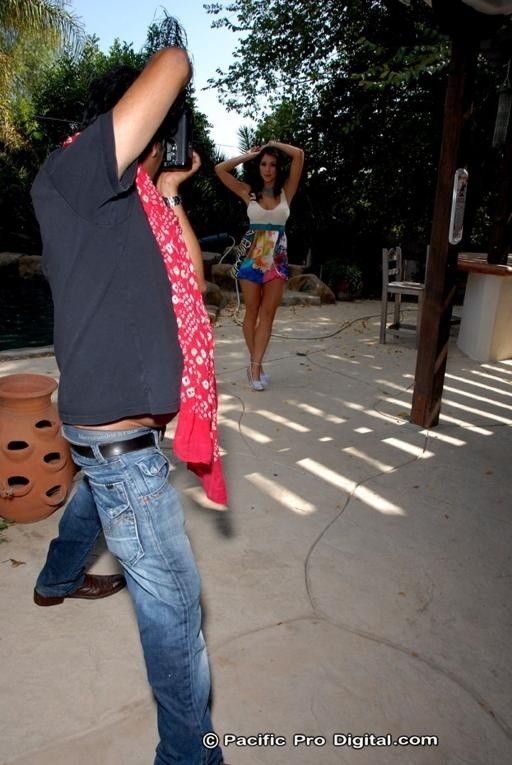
380,246 -> 425,343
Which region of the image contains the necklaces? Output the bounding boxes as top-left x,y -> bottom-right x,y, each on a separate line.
261,188 -> 276,195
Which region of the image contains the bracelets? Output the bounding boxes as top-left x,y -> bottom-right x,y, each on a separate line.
161,194 -> 184,208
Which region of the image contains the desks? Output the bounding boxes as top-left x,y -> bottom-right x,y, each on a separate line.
454,259 -> 512,363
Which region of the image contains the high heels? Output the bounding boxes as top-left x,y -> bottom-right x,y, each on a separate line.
247,360 -> 268,391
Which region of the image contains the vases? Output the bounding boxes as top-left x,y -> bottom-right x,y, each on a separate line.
0,374 -> 79,524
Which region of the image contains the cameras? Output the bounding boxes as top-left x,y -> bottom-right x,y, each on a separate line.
140,88 -> 194,169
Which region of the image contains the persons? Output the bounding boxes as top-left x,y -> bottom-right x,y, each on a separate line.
31,44 -> 232,765
214,141 -> 304,392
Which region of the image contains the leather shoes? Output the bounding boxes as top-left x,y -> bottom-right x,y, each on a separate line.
34,572 -> 127,606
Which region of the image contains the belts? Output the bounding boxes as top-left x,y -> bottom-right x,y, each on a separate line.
71,428 -> 167,459
